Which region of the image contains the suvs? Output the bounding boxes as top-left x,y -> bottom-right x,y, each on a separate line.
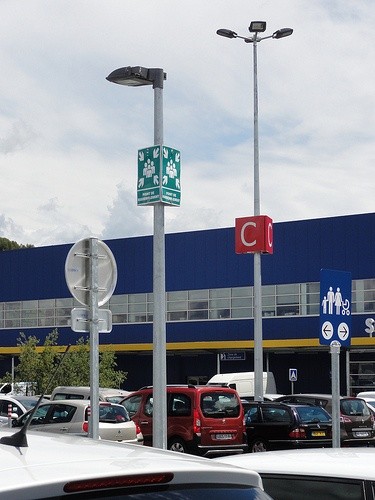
233,399 -> 334,453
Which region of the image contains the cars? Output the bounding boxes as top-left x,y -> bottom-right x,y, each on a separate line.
0,424 -> 274,500
3,399 -> 145,445
272,391 -> 375,447
213,446 -> 375,500
0,395 -> 50,429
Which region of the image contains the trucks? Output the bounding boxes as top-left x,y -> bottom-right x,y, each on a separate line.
0,381 -> 38,396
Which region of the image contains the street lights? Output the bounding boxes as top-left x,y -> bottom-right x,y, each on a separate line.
215,19 -> 293,403
104,65 -> 168,448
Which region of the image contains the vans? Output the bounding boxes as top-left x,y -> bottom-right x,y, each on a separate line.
50,386 -> 133,403
116,383 -> 247,457
203,371 -> 277,402
